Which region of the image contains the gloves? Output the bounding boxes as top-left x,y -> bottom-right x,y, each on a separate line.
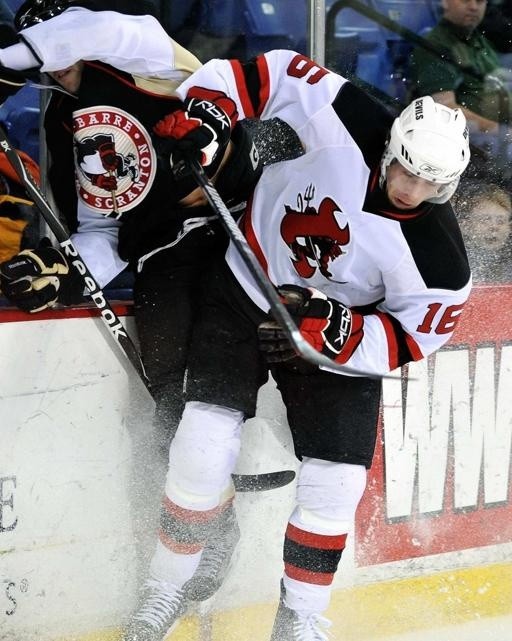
258,297 -> 365,361
0,247 -> 70,314
152,85 -> 239,175
0,50 -> 25,106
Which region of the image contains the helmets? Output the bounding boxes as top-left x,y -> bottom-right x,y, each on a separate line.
379,96 -> 471,205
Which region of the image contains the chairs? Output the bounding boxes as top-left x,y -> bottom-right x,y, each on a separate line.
1,0 -> 512,161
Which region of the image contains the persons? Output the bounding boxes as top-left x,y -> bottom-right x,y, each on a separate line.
114,48 -> 472,641
0,0 -> 512,493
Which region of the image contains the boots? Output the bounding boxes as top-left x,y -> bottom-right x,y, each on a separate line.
182,503 -> 241,601
266,576 -> 330,641
119,580 -> 185,641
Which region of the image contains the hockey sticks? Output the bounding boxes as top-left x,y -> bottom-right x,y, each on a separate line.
0,121 -> 294,492
190,162 -> 418,382
